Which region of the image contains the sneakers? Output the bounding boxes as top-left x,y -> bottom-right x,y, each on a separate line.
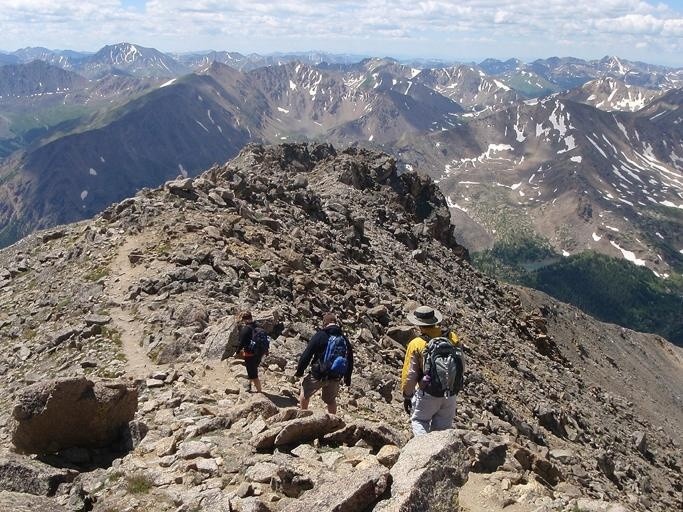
244,383 -> 251,392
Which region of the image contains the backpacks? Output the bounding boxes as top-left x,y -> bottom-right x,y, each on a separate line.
420,330 -> 464,398
320,331 -> 349,380
246,325 -> 268,356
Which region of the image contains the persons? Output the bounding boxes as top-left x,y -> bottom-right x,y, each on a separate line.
232,311 -> 270,394
400,306 -> 465,438
292,312 -> 353,415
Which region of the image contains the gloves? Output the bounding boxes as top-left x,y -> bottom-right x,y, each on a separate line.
404,399 -> 412,414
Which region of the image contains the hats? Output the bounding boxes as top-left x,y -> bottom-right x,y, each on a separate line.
408,306 -> 443,326
322,312 -> 336,327
